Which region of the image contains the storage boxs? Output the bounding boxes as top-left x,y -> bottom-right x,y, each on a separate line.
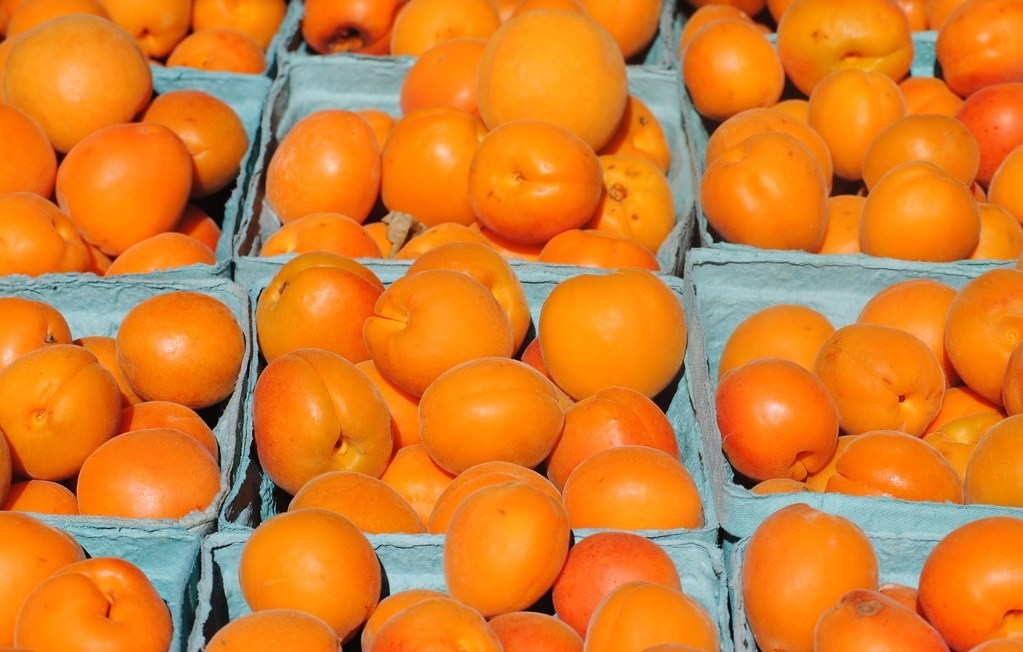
0,0 -> 1023,652
